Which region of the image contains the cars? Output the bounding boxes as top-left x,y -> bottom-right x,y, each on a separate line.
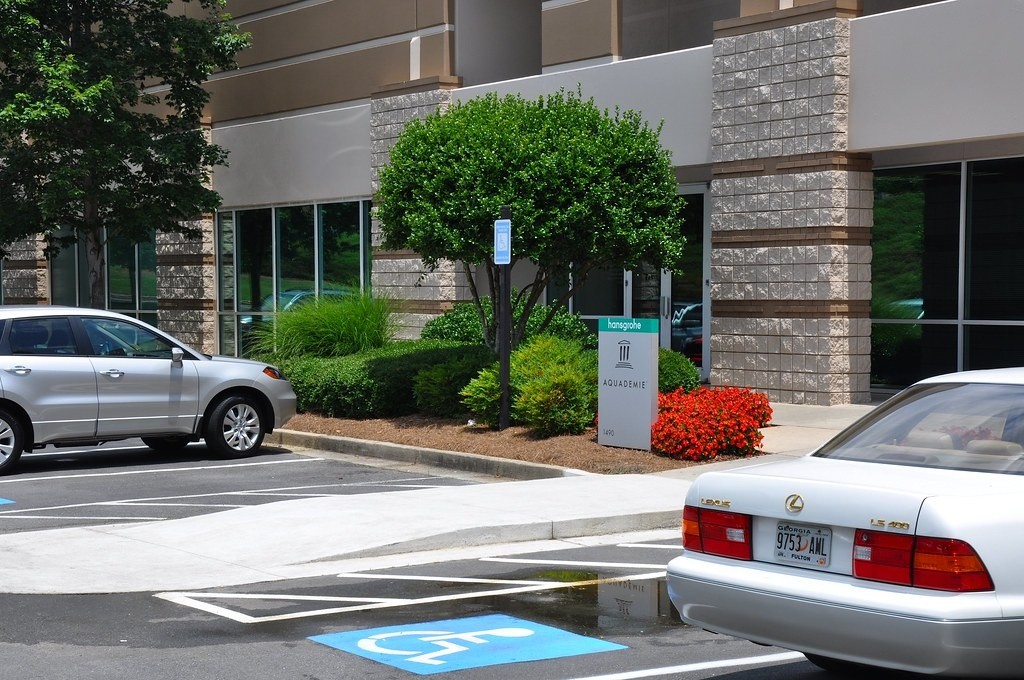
665,365 -> 1024,680
667,299 -> 705,366
887,298 -> 924,335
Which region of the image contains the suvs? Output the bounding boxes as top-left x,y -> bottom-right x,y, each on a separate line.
0,304 -> 299,476
240,287 -> 361,343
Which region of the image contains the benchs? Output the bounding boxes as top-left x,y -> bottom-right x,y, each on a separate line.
875,432 -> 1024,462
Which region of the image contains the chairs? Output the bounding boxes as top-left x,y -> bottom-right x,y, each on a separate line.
16,326 -> 49,353
